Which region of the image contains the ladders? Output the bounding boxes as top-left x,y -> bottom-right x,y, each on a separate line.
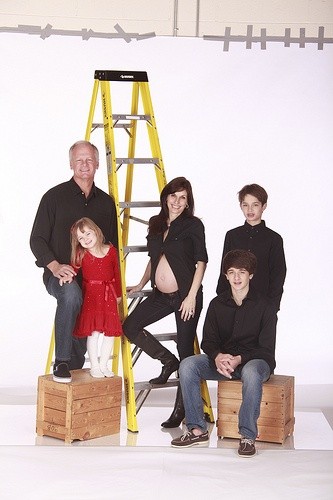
46,70 -> 216,432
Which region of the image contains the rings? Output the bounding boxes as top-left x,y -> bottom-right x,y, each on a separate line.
189,312 -> 193,315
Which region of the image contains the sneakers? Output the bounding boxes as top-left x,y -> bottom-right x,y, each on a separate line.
53,364 -> 72,383
237,436 -> 257,458
170,430 -> 210,448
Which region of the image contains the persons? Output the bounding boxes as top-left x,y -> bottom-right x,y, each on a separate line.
58,217 -> 123,379
171,249 -> 275,458
30,139 -> 119,383
122,177 -> 209,428
216,184 -> 288,325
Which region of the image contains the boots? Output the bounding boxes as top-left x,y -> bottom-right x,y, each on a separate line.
87,331 -> 105,378
133,328 -> 180,384
98,336 -> 114,378
161,382 -> 185,428
